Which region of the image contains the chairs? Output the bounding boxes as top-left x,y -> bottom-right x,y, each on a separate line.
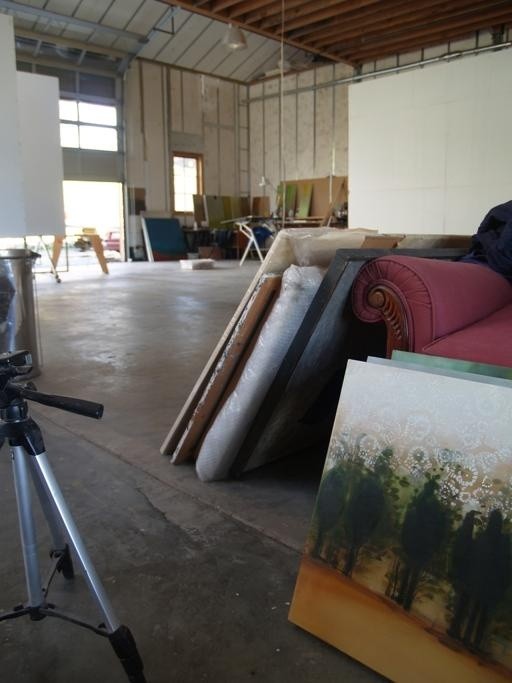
350,254 -> 511,369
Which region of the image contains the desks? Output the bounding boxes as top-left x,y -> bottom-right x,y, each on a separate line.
182,227 -> 213,251
214,228 -> 250,260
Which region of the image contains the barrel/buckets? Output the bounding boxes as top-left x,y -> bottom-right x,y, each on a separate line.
0,249 -> 41,383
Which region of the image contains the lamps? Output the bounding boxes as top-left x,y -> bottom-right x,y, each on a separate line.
222,22 -> 247,51
259,176 -> 282,219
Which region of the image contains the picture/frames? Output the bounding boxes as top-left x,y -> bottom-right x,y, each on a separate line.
228,247 -> 471,480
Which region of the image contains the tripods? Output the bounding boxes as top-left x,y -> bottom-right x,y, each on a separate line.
0,349 -> 146,678
